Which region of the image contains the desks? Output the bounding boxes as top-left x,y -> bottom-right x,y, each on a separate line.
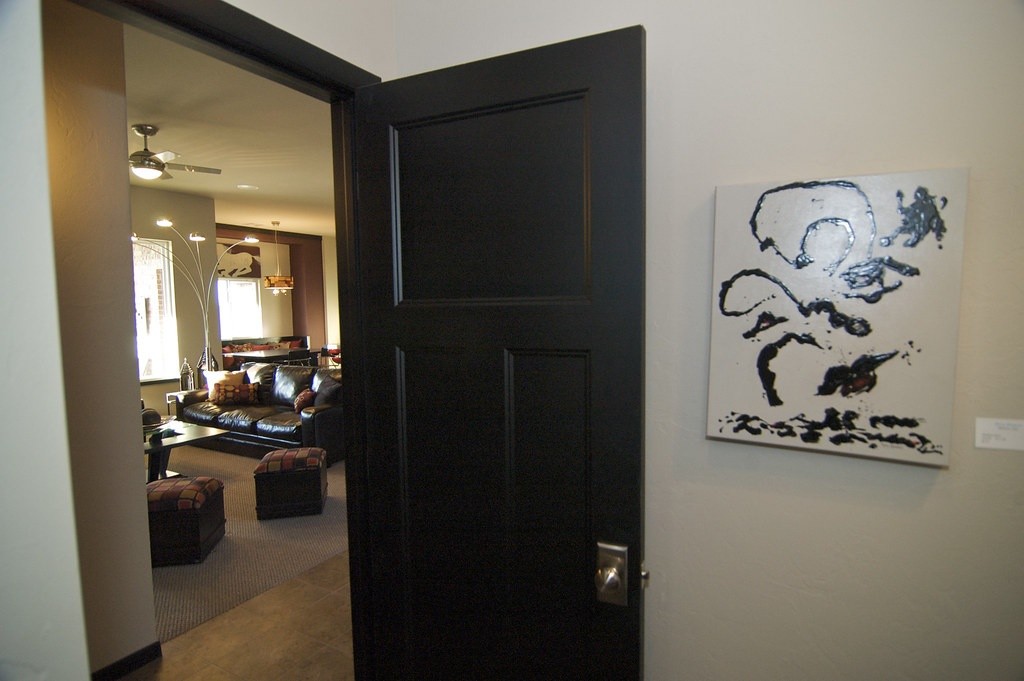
234,348 -> 320,368
143,420 -> 231,482
166,389 -> 197,415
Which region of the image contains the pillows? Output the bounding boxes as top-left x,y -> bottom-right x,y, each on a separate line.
213,383 -> 260,407
294,388 -> 317,413
203,370 -> 245,401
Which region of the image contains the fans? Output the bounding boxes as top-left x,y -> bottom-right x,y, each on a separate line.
129,123 -> 221,181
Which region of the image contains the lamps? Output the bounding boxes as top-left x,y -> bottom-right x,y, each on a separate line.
265,221 -> 293,295
191,232 -> 205,242
157,217 -> 172,227
244,234 -> 259,243
132,168 -> 162,180
131,232 -> 139,242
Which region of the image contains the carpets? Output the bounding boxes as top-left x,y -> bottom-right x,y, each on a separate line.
142,446 -> 348,644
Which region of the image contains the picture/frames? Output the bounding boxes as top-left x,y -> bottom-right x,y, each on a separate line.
706,168 -> 965,467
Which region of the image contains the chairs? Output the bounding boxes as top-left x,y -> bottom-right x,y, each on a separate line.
288,350 -> 310,366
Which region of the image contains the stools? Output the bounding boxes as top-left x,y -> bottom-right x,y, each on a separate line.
146,477 -> 226,567
254,447 -> 328,519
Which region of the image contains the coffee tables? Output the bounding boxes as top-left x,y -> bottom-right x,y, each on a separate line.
176,362 -> 345,468
